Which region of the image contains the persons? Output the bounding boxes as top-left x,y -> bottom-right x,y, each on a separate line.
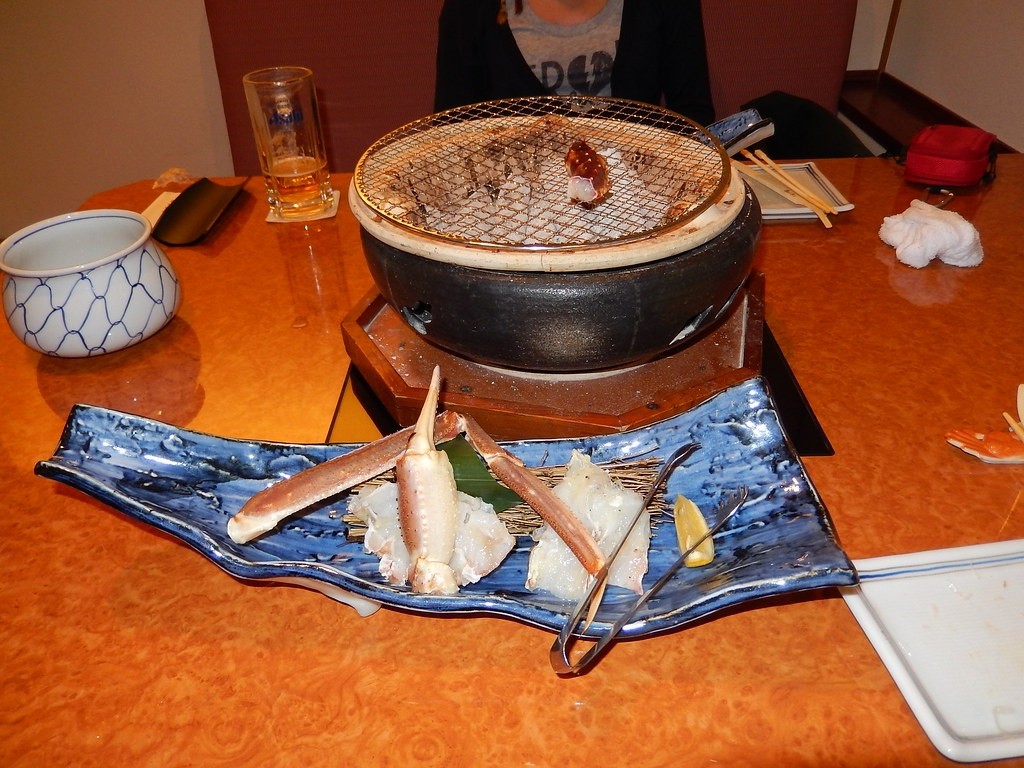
433,0 -> 715,136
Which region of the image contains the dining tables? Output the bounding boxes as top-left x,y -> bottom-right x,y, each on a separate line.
0,152 -> 1024,768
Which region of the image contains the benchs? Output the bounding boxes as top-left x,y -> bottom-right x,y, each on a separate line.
202,0 -> 857,175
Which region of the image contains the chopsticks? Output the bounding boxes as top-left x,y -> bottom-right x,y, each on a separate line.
740,150 -> 837,215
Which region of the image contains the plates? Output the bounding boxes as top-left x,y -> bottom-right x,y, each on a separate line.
692,109 -> 774,158
837,539 -> 1024,763
34,376 -> 858,638
738,162 -> 854,219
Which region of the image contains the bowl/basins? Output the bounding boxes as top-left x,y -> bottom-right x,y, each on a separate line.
0,209 -> 180,357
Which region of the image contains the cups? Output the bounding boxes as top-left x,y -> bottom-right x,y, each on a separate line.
243,66 -> 334,221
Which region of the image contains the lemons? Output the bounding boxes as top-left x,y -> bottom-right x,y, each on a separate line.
673,494 -> 714,567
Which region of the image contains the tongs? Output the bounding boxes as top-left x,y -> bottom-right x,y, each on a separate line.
550,443 -> 749,673
